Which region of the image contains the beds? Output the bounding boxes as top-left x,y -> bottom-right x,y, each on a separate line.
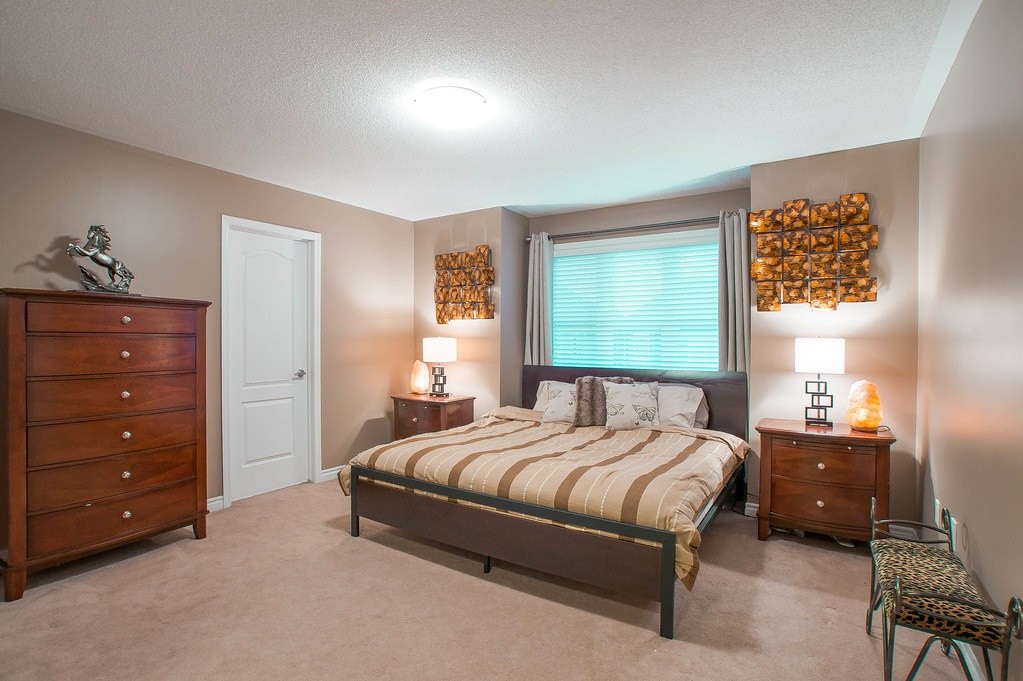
336,365 -> 755,639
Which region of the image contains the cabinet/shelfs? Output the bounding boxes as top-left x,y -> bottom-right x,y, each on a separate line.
0,285 -> 217,604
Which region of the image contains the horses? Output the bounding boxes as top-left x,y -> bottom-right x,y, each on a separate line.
66,224 -> 135,289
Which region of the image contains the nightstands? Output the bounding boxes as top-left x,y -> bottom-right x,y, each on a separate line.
756,418 -> 898,554
390,393 -> 475,438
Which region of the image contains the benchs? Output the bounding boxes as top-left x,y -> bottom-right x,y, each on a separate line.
868,496 -> 1022,680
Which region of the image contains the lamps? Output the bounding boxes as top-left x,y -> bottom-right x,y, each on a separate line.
423,336 -> 457,398
792,338 -> 847,428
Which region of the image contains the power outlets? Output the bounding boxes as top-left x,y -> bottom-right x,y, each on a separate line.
948,516 -> 959,554
932,499 -> 940,526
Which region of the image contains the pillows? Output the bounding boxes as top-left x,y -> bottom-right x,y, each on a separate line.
631,382 -> 710,429
603,380 -> 661,430
572,375 -> 635,427
534,380 -> 577,412
650,386 -> 704,428
541,389 -> 584,421
